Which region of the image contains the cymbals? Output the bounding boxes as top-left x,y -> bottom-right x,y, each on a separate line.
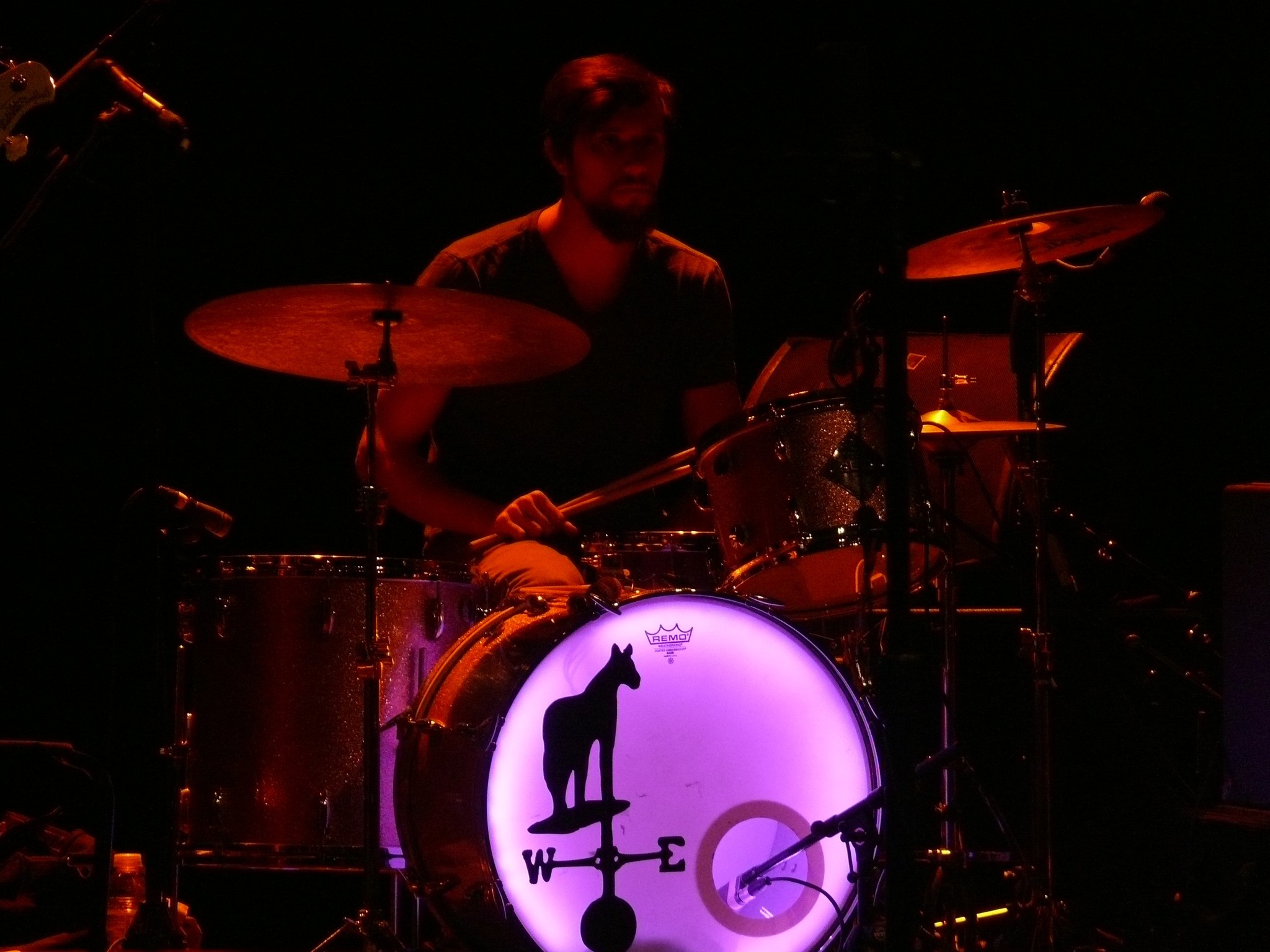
915,408 -> 1068,470
185,281 -> 592,387
900,202 -> 1170,282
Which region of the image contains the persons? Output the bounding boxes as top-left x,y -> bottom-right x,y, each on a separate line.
355,51 -> 746,615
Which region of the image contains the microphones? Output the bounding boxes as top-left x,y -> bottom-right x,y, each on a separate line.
1090,191 -> 1171,269
724,877 -> 772,910
827,290 -> 874,376
154,484 -> 233,538
54,35 -> 116,90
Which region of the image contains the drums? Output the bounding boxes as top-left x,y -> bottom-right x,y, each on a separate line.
162,551 -> 476,876
386,590 -> 885,952
695,385 -> 946,619
580,529 -> 726,609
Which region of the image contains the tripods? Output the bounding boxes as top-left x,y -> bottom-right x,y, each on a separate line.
312,320 -> 403,951
906,235 -> 1126,952
